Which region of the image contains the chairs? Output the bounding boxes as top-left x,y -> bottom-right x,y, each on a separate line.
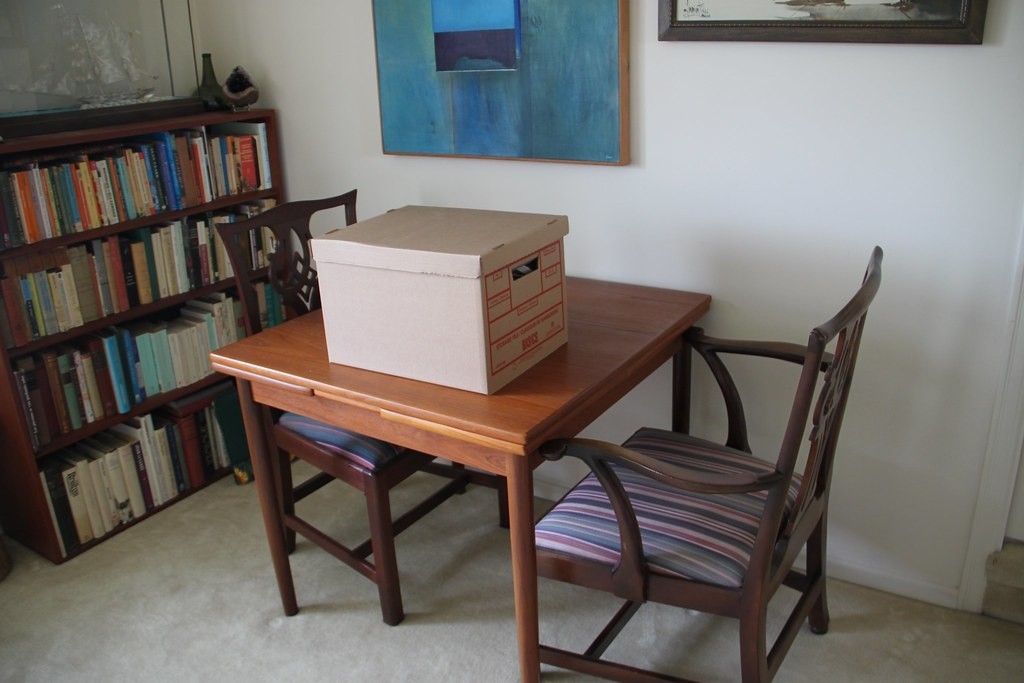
212,188 -> 510,628
533,246 -> 884,683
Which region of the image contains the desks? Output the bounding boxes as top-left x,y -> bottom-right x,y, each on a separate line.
208,273 -> 711,683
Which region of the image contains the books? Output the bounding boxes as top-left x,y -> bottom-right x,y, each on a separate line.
0,122 -> 271,249
10,279 -> 288,451
1,199 -> 279,348
36,381 -> 249,559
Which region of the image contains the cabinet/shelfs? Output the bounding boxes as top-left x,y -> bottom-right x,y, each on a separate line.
1,100 -> 289,565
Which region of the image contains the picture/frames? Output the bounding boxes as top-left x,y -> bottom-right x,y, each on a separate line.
656,1 -> 991,45
372,1 -> 632,168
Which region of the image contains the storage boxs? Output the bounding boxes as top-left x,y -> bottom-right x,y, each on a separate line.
983,550 -> 1024,623
309,205 -> 571,396
